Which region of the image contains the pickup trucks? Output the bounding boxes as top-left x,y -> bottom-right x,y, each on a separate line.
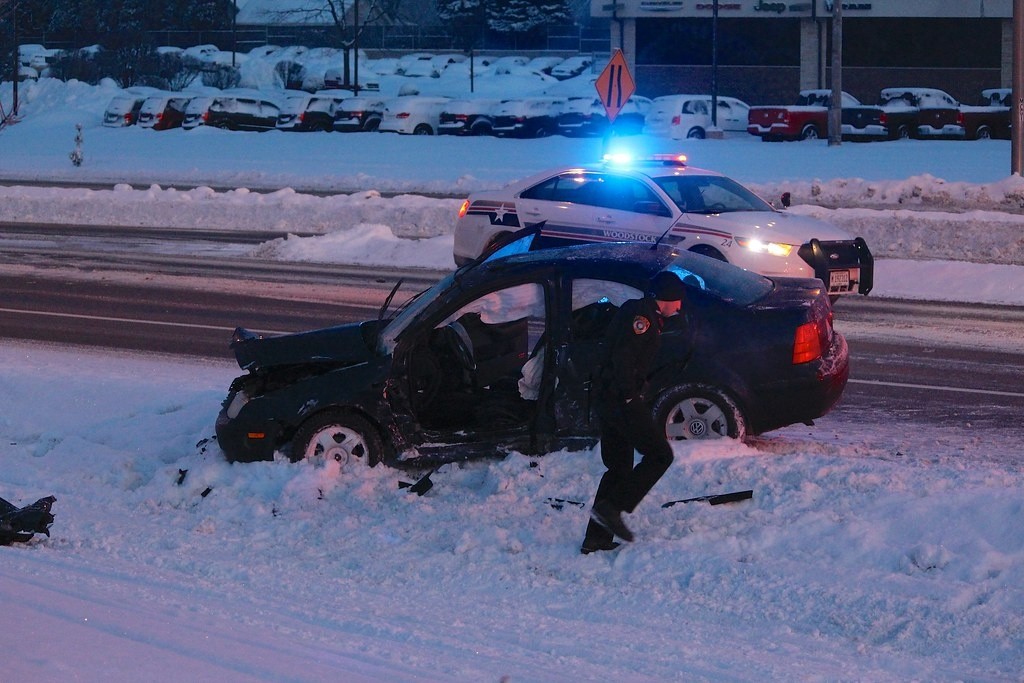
747,87 -> 1012,143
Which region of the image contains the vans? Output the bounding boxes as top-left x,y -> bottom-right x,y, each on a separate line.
101,86 -> 747,139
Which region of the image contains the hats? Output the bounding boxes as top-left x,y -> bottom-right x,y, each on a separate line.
644,270 -> 686,301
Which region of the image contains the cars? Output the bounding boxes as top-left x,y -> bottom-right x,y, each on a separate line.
215,218 -> 851,471
17,44 -> 592,77
454,154 -> 874,307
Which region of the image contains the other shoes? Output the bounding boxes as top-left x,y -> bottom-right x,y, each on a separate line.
591,502 -> 635,543
582,535 -> 620,555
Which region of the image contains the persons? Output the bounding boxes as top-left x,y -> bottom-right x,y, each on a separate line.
582,272 -> 683,554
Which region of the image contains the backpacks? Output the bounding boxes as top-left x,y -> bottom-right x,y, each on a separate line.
564,300 -> 633,383
